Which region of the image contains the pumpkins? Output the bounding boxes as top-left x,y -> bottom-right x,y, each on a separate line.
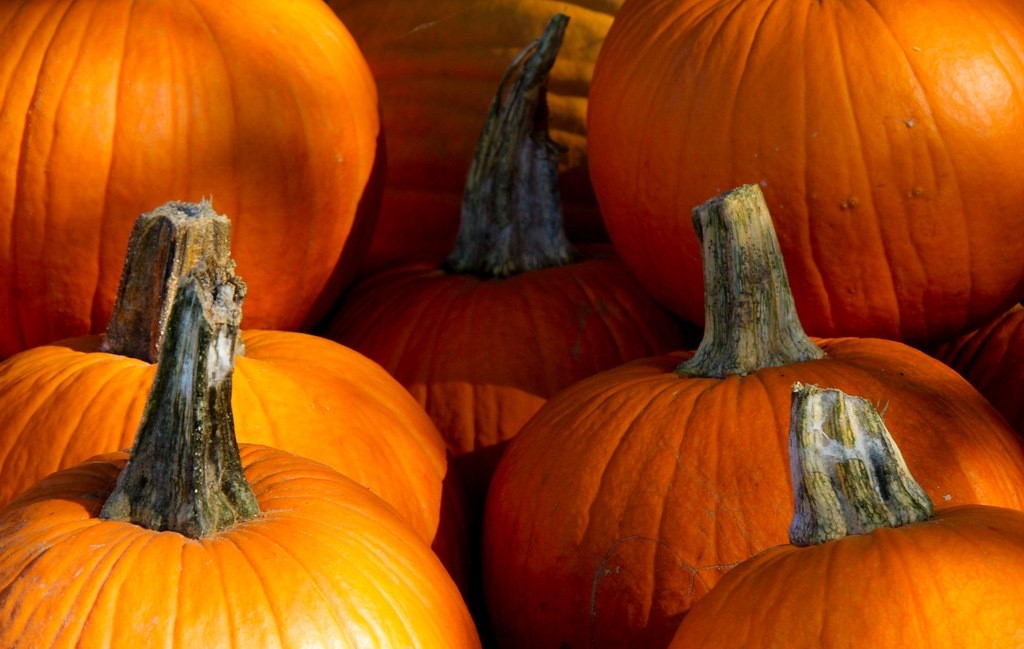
0,0 -> 1024,649
